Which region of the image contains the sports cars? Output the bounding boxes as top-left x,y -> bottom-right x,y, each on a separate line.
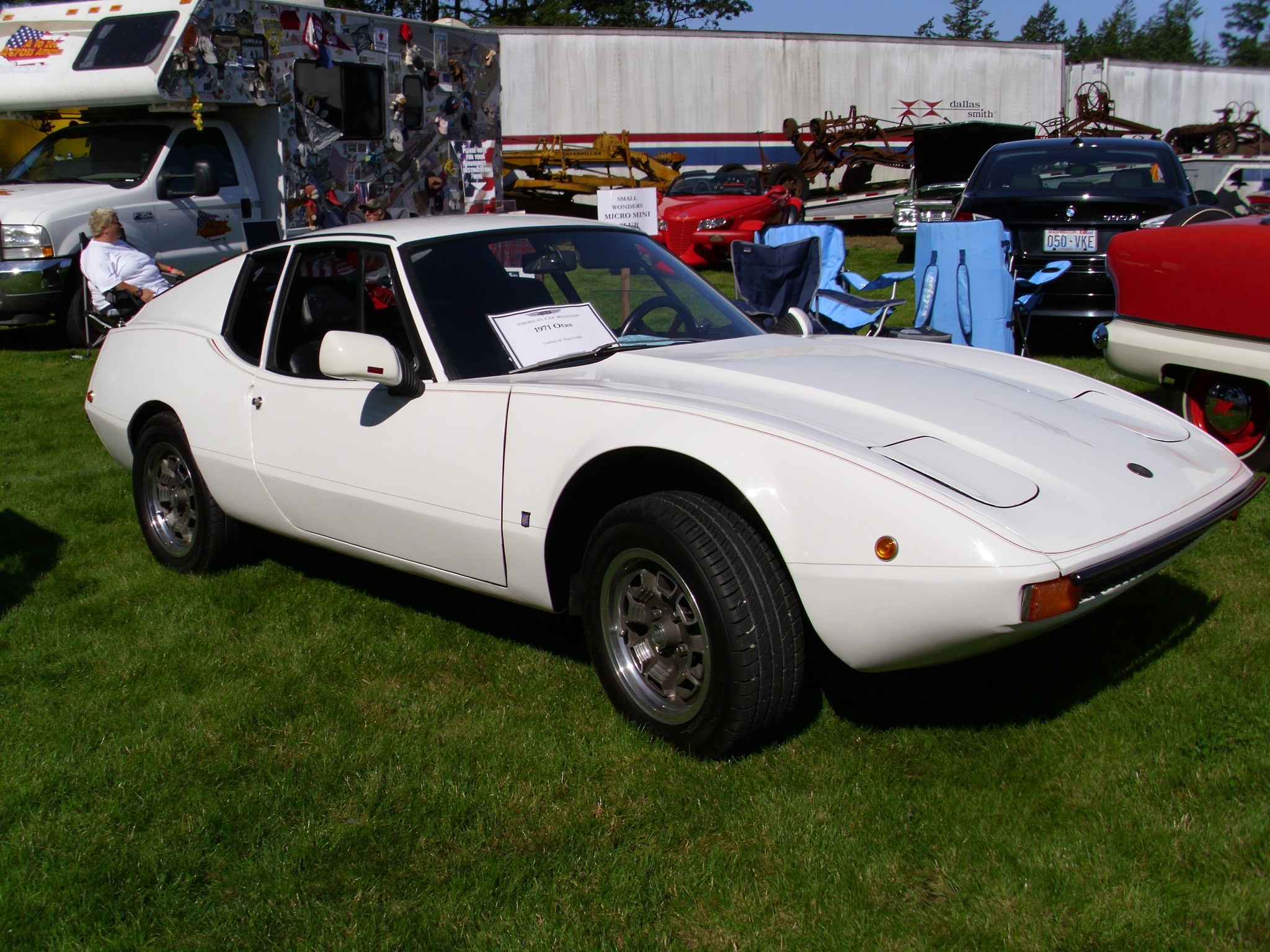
638,168 -> 806,268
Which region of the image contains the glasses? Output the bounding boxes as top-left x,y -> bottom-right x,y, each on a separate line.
362,207 -> 376,214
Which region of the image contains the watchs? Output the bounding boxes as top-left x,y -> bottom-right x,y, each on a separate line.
134,286 -> 144,299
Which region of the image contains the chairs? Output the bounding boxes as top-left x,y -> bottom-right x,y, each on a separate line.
1109,169 -> 1148,191
1011,173 -> 1042,191
347,209 -> 409,224
79,230 -> 185,358
731,237 -> 907,338
288,283 -> 368,382
752,224 -> 916,337
241,220 -> 280,252
912,219 -> 1073,358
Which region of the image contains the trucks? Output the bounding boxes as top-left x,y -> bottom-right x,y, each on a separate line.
0,0 -> 504,347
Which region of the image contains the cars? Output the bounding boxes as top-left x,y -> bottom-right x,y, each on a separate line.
1090,197 -> 1270,478
944,135 -> 1217,347
1155,153 -> 1270,205
891,120 -> 1036,254
86,209 -> 1270,760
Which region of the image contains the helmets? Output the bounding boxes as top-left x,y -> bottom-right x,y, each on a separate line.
272,31 -> 276,34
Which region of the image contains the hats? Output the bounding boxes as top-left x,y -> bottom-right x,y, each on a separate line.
257,59 -> 272,82
423,66 -> 439,92
424,171 -> 443,198
439,170 -> 448,187
390,128 -> 404,151
325,187 -> 345,207
358,198 -> 385,210
198,36 -> 219,64
249,78 -> 268,106
448,58 -> 463,82
462,91 -> 473,111
433,190 -> 445,212
467,46 -> 480,68
445,95 -> 460,114
321,10 -> 336,33
401,22 -> 414,42
484,104 -> 496,124
410,157 -> 421,183
405,42 -> 430,71
298,142 -> 314,167
461,112 -> 473,132
435,113 -> 449,135
412,188 -> 430,214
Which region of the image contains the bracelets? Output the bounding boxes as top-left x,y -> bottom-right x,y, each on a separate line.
167,266 -> 173,274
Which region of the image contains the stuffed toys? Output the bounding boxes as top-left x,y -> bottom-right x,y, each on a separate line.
305,185 -> 320,231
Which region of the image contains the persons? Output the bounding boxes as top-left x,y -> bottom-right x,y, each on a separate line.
80,206 -> 186,317
358,199 -> 386,223
